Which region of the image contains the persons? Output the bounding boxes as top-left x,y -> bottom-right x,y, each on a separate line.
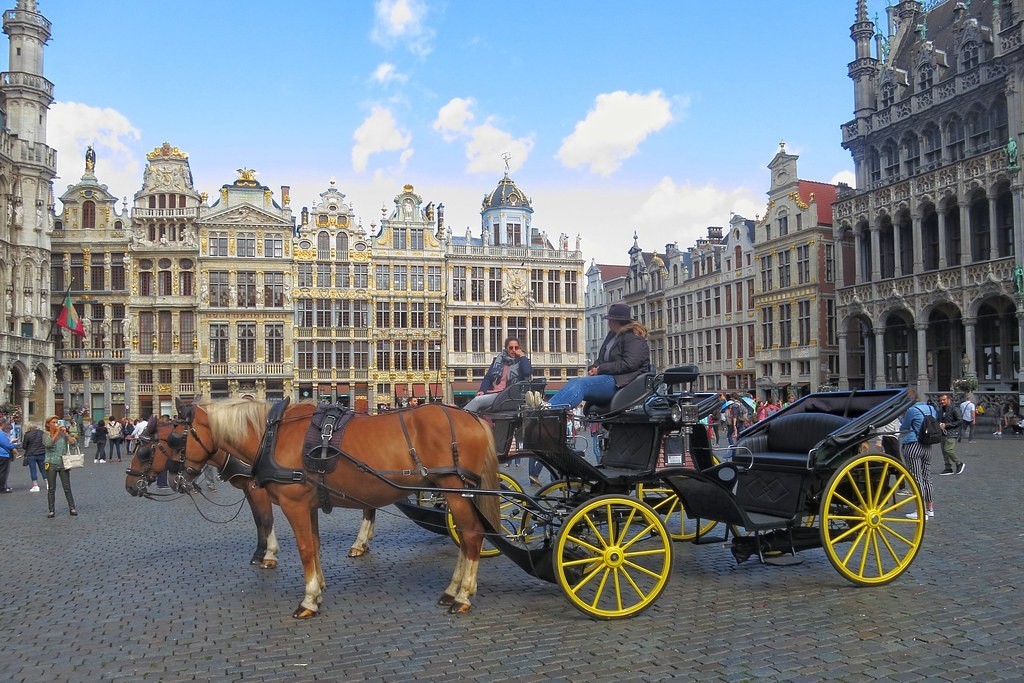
463,336 -> 532,413
699,392 -> 795,448
192,464 -> 218,494
0,418 -> 19,494
446,226 -> 582,250
68,419 -> 93,448
6,291 -> 46,316
503,458 -> 542,486
579,404 -> 584,428
1002,400 -> 1012,429
7,202 -> 53,231
408,397 -> 446,502
875,389 -> 936,521
958,395 -> 976,444
405,200 -> 412,219
7,367 -> 56,383
22,422 -> 49,492
938,394 -> 965,475
993,400 -> 1002,435
525,304 -> 650,411
61,314 -> 130,340
41,415 -> 78,518
93,414 -> 178,490
1012,416 -> 1024,435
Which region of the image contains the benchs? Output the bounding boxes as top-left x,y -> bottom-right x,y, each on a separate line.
733,411 -> 854,471
483,378 -> 547,410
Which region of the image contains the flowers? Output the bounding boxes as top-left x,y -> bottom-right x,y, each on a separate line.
951,378 -> 977,393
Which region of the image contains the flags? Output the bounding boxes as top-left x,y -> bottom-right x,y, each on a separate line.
57,293 -> 86,339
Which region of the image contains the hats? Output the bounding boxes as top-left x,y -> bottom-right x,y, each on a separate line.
603,303 -> 634,322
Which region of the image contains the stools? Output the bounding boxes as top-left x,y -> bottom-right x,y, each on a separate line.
584,365 -> 655,416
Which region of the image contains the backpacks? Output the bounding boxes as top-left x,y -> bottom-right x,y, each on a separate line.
911,404 -> 943,445
22,455 -> 29,466
735,403 -> 748,422
108,421 -> 122,440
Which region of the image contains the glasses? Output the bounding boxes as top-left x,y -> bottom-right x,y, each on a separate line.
508,345 -> 519,350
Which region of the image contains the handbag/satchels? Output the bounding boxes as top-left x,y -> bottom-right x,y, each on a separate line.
93,434 -> 97,443
62,438 -> 85,470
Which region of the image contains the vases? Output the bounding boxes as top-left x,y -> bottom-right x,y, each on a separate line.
959,385 -> 969,392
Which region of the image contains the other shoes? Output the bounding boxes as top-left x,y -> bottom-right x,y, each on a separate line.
29,486 -> 41,493
992,432 -> 1002,435
108,459 -> 113,464
939,468 -> 955,476
712,444 -> 719,448
209,483 -> 218,494
906,507 -> 935,521
69,509 -> 78,516
46,485 -> 49,490
94,459 -> 106,464
192,488 -> 199,494
896,489 -> 916,497
48,511 -> 55,518
529,475 -> 543,488
117,458 -> 122,463
523,391 -> 551,410
956,462 -> 965,475
157,485 -> 171,490
0,488 -> 13,493
968,440 -> 977,444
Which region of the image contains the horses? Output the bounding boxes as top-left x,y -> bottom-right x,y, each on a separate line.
126,393 -> 500,619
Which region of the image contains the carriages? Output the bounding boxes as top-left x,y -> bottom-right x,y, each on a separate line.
123,364 -> 928,621
